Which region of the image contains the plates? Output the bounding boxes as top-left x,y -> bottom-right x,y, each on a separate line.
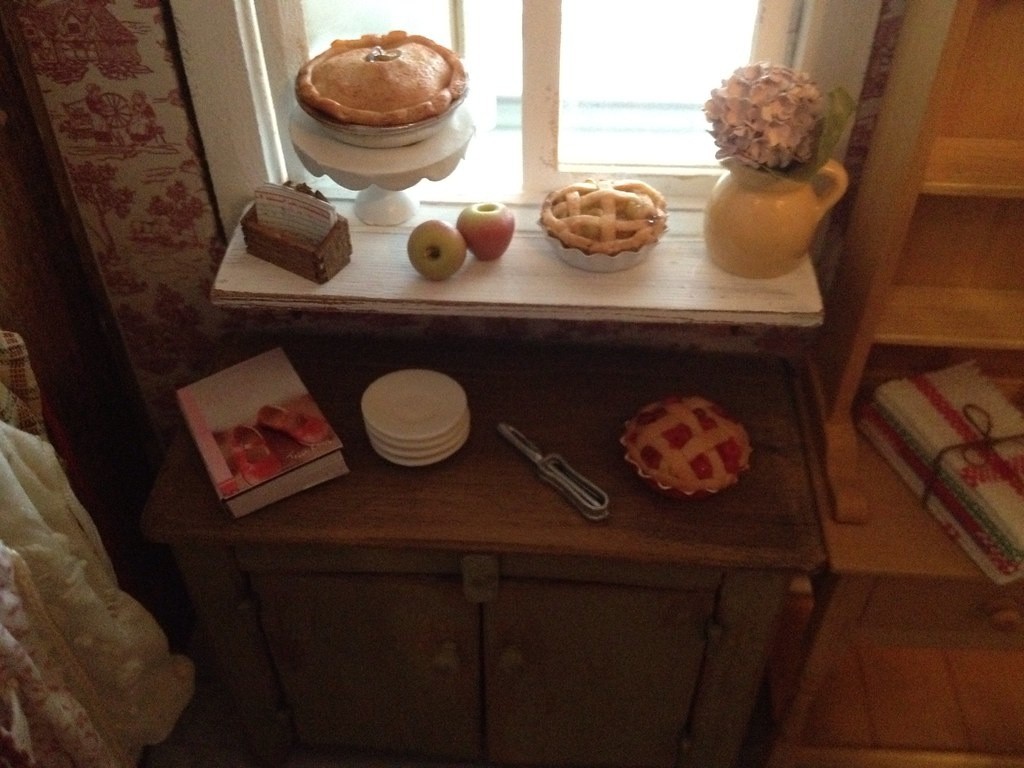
361,369 -> 470,467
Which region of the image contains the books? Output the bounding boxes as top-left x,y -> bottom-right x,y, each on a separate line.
176,347 -> 348,520
856,355 -> 1024,585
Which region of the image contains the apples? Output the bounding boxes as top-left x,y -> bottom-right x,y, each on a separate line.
456,202 -> 515,260
407,220 -> 466,280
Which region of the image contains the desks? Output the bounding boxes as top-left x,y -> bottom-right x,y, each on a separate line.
756,377 -> 1024,768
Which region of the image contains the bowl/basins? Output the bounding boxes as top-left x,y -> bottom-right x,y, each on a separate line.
537,218 -> 668,272
293,80 -> 468,149
619,419 -> 751,501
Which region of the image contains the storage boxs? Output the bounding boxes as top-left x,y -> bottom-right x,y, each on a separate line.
857,361 -> 1024,583
239,179 -> 352,286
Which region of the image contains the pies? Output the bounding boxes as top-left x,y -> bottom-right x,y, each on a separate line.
622,392 -> 751,493
300,30 -> 466,127
536,177 -> 668,272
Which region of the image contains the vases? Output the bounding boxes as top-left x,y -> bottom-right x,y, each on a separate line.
702,157 -> 848,279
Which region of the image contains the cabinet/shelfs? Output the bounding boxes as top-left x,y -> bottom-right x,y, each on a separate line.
800,0 -> 1024,525
137,203 -> 824,768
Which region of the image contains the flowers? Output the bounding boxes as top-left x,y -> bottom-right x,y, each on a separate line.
702,62 -> 855,183
